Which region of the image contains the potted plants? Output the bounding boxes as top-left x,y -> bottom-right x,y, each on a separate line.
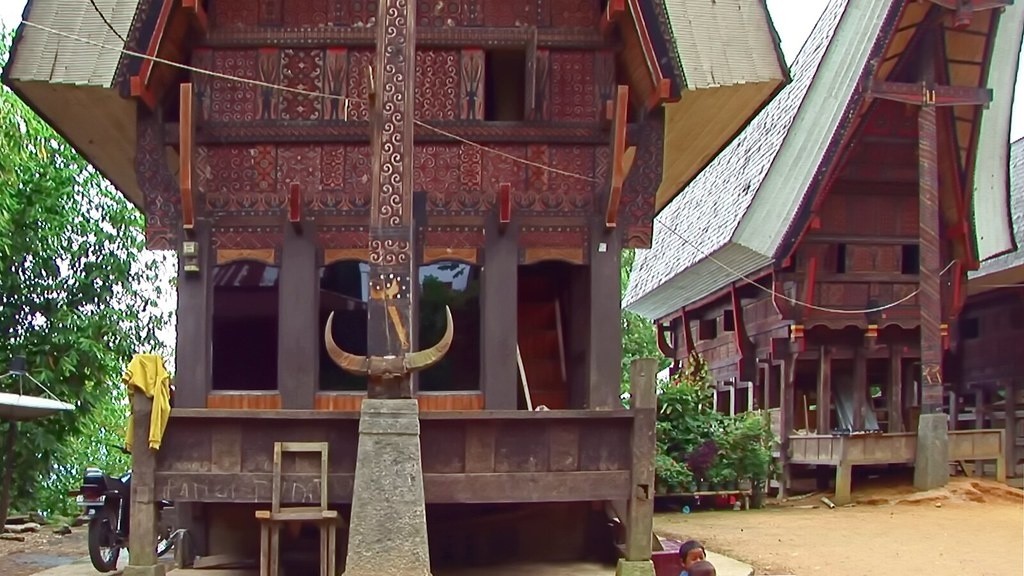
656,440 -> 754,493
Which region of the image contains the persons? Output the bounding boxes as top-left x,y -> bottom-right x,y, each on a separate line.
687,560 -> 716,576
678,541 -> 706,576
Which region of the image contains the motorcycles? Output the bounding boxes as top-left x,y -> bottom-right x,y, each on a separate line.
77,444 -> 172,573
